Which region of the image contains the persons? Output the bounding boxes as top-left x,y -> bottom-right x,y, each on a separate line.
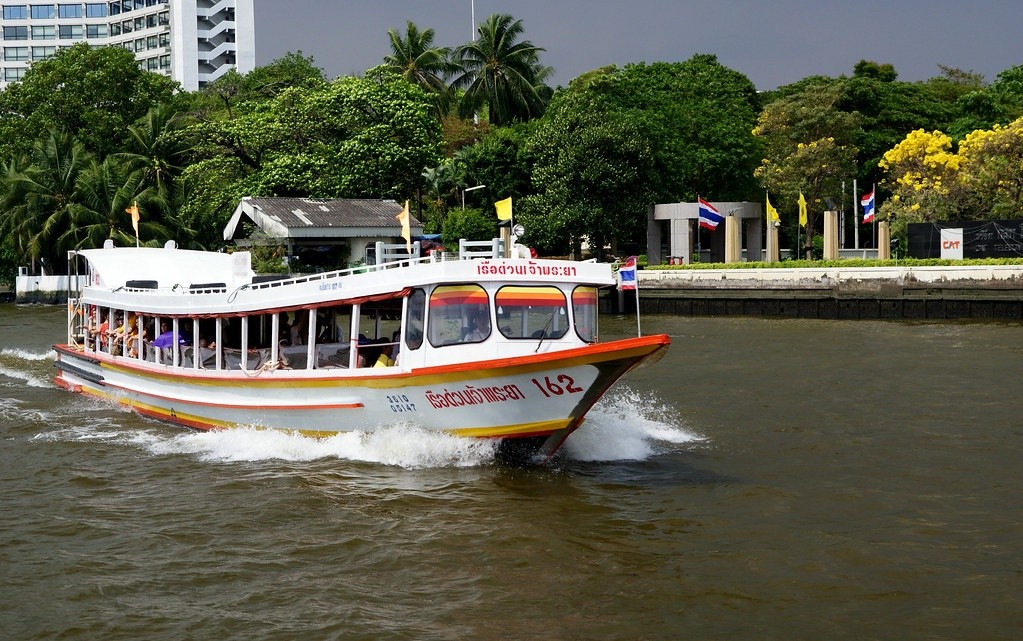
464,310 -> 490,342
420,240 -> 443,263
75,305 -> 421,369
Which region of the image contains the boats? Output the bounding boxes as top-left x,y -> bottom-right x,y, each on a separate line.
49,239 -> 670,466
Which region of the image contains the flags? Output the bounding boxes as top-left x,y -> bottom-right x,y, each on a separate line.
617,260 -> 636,290
861,192 -> 873,223
699,198 -> 722,230
766,198 -> 781,222
494,197 -> 512,221
396,206 -> 410,252
798,194 -> 807,226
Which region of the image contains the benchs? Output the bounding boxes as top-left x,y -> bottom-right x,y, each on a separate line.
125,338 -> 396,370
